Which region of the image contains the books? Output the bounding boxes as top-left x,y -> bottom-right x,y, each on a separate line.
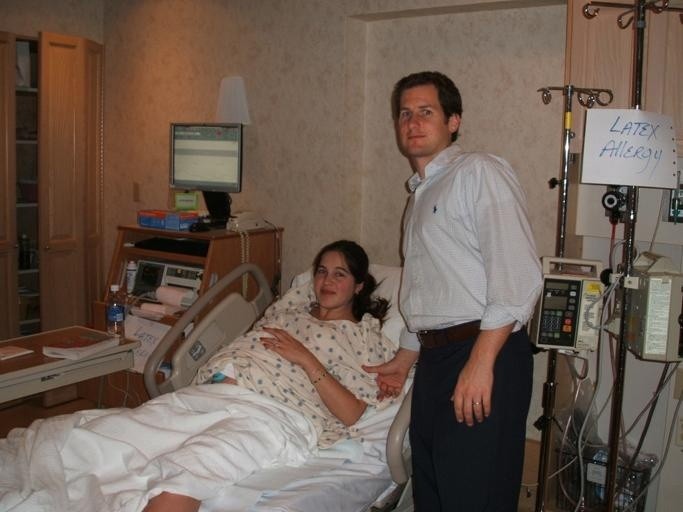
43,331 -> 119,360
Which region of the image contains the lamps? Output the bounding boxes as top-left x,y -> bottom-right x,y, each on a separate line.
216,76 -> 250,126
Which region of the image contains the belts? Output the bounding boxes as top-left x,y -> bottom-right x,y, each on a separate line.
414,318 -> 482,350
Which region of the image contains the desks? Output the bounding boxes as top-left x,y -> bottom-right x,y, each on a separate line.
0,325 -> 141,412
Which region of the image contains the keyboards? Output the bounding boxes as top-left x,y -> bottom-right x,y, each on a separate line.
134,237 -> 208,257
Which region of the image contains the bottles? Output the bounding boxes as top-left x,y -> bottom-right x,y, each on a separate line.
18,233 -> 30,269
126,261 -> 138,298
106,284 -> 124,345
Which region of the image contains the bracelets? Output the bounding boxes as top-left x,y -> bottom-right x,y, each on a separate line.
312,371 -> 329,385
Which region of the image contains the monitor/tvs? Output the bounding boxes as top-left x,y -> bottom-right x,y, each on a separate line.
169,121 -> 243,230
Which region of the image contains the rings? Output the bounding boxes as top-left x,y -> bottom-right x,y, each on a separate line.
473,402 -> 483,405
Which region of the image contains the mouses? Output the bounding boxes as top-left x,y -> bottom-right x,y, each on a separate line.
189,223 -> 210,232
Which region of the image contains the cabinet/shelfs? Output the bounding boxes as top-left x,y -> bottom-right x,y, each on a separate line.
88,224 -> 285,407
533,1 -> 683,512
0,30 -> 106,339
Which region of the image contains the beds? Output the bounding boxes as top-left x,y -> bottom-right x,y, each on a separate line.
0,261 -> 416,512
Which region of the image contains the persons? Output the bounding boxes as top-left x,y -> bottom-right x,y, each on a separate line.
141,240 -> 398,512
362,71 -> 544,512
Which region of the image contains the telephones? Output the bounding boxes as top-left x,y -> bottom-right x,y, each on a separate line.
226,210 -> 264,231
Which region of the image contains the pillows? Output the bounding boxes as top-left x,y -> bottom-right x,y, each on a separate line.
292,264 -> 402,350
